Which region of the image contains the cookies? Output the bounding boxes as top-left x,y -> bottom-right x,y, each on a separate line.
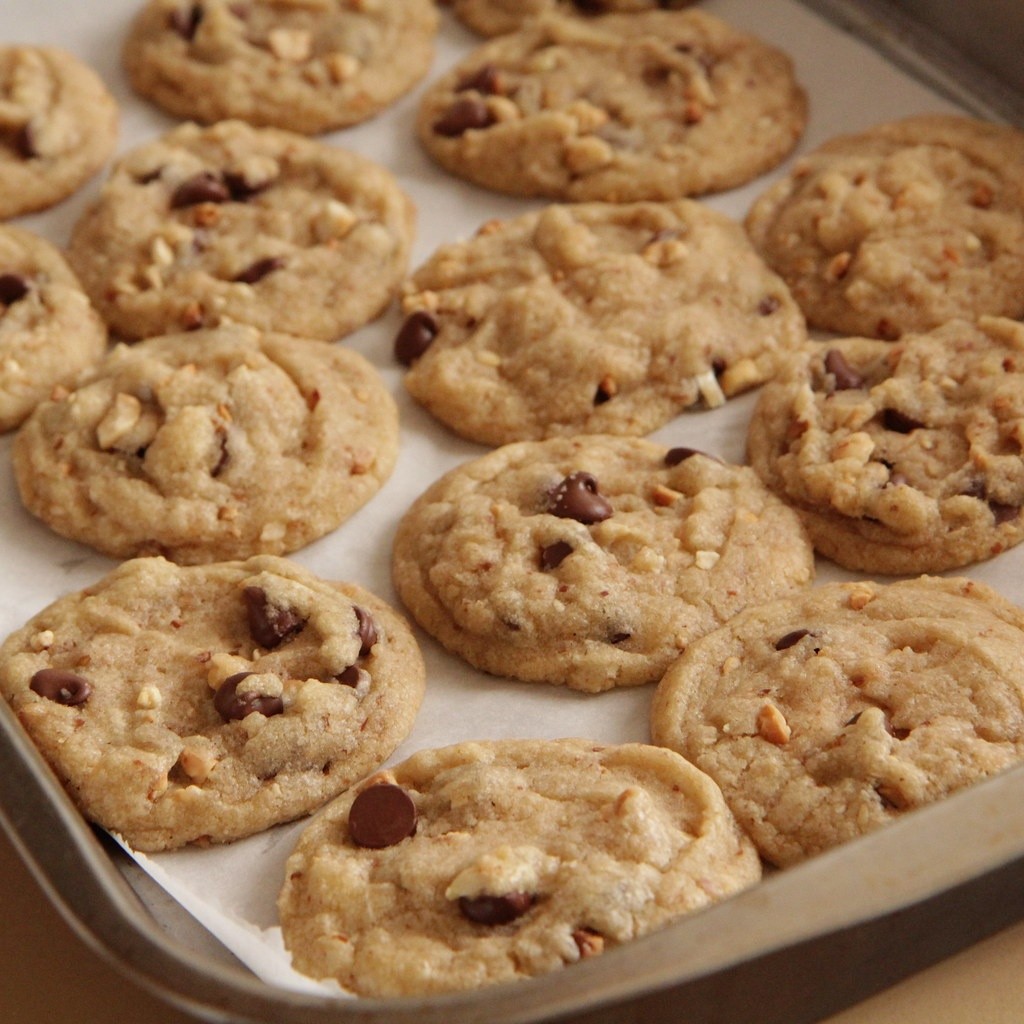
0,0 -> 1023,1001
